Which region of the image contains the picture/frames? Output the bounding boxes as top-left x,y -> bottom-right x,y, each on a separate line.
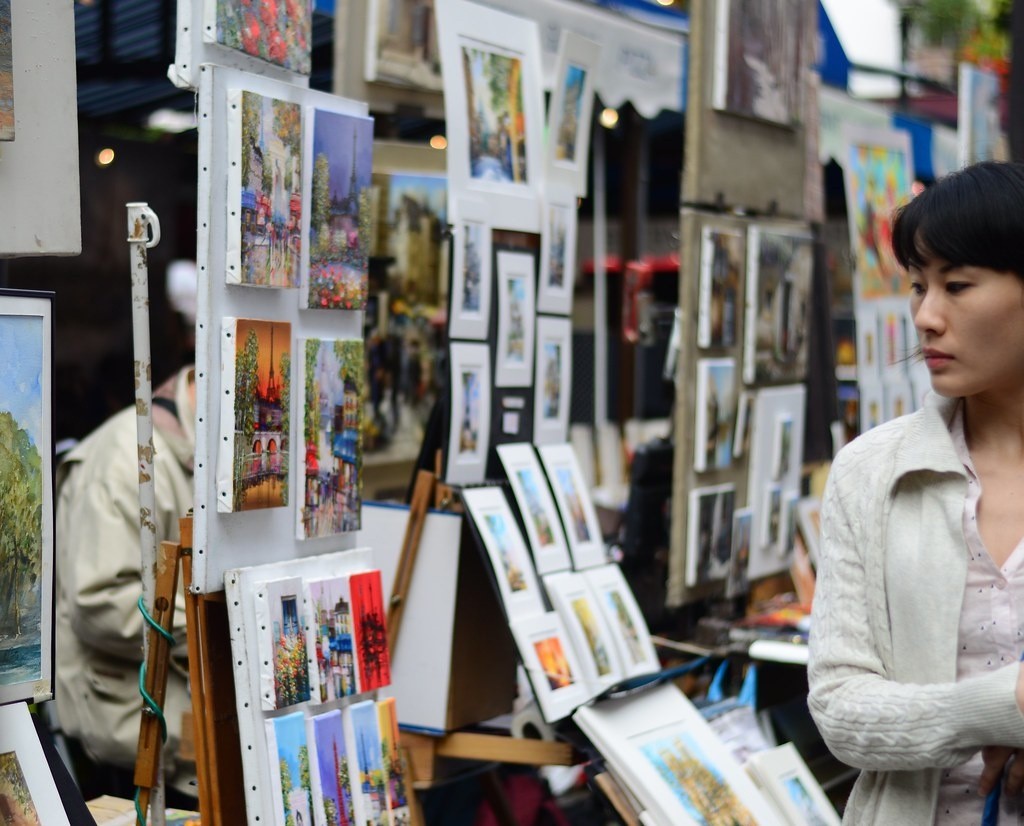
0,702 -> 71,826
0,286 -> 61,705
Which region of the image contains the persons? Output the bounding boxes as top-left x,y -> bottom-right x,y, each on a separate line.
808,160 -> 1023,826
54,369 -> 197,809
